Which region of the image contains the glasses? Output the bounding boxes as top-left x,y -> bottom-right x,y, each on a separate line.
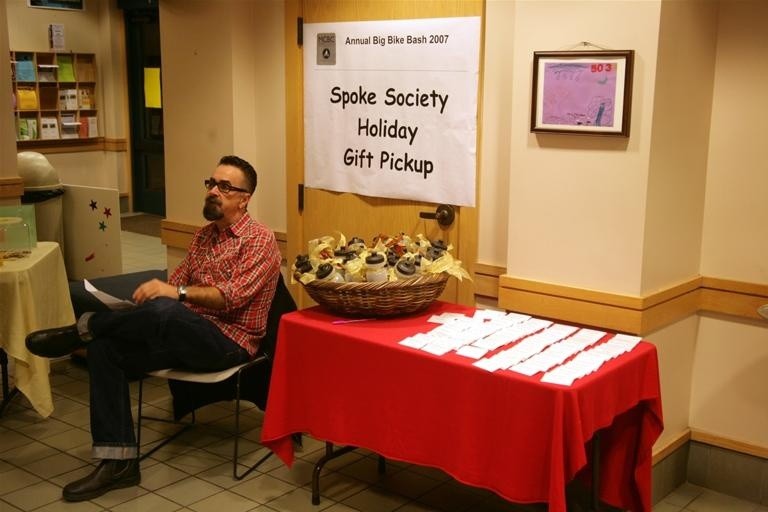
205,179 -> 247,194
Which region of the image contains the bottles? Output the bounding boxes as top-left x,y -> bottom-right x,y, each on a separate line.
292,237 -> 448,282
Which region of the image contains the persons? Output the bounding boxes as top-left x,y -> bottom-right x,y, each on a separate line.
26,156 -> 283,500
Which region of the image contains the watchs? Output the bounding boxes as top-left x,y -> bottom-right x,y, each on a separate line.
178,285 -> 187,302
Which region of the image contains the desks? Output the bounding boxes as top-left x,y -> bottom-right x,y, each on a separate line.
0,240 -> 78,418
260,294 -> 664,512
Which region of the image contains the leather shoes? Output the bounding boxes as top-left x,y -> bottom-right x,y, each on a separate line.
25,324 -> 87,358
63,458 -> 141,502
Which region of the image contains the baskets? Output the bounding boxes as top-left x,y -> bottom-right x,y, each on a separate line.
294,261 -> 451,318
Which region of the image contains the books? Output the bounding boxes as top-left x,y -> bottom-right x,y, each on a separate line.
15,54 -> 102,141
49,22 -> 69,52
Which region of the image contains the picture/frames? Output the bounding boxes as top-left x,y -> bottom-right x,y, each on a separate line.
528,50 -> 635,138
26,0 -> 86,13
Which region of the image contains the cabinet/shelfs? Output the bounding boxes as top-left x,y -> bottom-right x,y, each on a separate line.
8,53 -> 101,142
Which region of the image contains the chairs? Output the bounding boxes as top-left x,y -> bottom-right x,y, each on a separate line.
135,271 -> 301,479
